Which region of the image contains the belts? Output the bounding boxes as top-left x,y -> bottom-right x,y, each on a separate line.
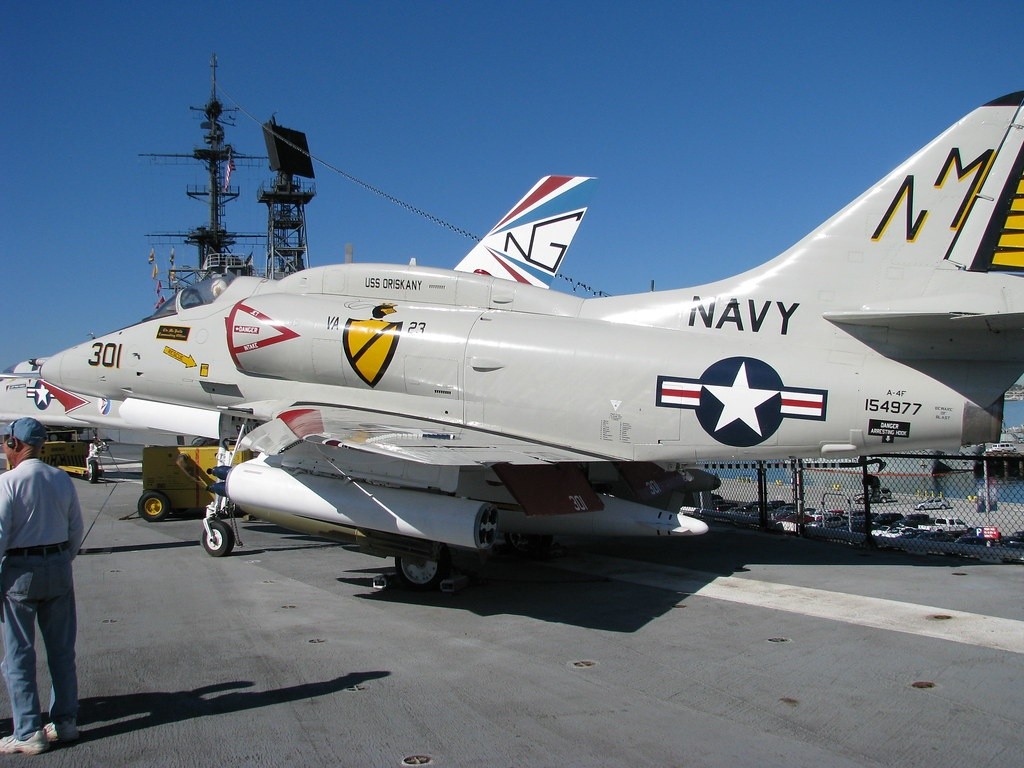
5,542 -> 68,558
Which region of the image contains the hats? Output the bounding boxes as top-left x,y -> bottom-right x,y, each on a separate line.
5,417 -> 47,448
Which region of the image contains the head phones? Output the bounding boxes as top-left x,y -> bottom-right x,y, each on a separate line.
7,419 -> 18,448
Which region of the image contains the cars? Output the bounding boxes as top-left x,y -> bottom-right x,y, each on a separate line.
710,493 -> 1024,560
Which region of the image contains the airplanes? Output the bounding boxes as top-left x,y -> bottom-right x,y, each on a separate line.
0,89 -> 1024,595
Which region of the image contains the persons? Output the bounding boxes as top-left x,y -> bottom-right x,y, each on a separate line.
0,418 -> 85,755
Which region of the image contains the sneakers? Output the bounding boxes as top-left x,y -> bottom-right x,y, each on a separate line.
43,718 -> 79,744
0,728 -> 50,755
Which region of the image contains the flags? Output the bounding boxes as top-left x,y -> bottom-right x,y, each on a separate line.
149,247 -> 176,309
225,150 -> 236,192
